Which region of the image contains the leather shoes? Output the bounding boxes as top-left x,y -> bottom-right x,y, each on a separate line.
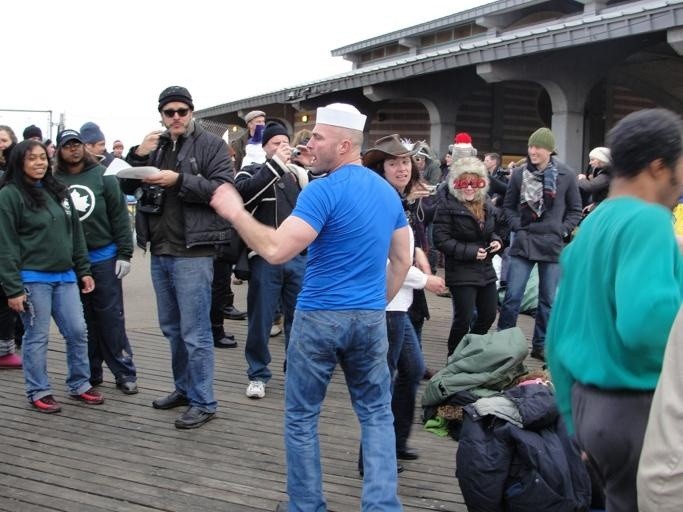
358,461 -> 404,475
224,334 -> 234,339
120,379 -> 138,395
150,391 -> 190,410
214,336 -> 237,348
395,448 -> 418,460
222,305 -> 246,321
173,404 -> 218,430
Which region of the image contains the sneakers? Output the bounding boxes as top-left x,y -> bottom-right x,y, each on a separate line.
276,500 -> 288,512
269,325 -> 281,336
69,388 -> 105,405
27,394 -> 62,414
0,352 -> 22,370
423,366 -> 441,380
528,349 -> 544,360
245,379 -> 266,399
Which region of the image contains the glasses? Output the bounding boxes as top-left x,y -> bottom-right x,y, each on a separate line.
62,142 -> 81,149
451,177 -> 486,191
161,106 -> 189,118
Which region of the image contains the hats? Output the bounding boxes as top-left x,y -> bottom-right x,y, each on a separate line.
78,122 -> 105,143
242,110 -> 266,126
313,101 -> 368,132
55,128 -> 84,148
446,156 -> 492,203
111,139 -> 124,149
22,124 -> 42,140
448,131 -> 478,155
156,85 -> 194,111
361,132 -> 424,168
260,122 -> 290,147
587,146 -> 612,163
409,140 -> 433,161
526,126 -> 558,153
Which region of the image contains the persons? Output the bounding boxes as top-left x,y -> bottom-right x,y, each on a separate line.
121,88 -> 314,428
358,128 -> 611,475
546,109 -> 682,511
1,120 -> 139,412
209,103 -> 411,511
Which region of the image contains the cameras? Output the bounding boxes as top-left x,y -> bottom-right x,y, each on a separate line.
484,246 -> 494,254
290,147 -> 301,155
424,185 -> 438,196
137,185 -> 167,216
